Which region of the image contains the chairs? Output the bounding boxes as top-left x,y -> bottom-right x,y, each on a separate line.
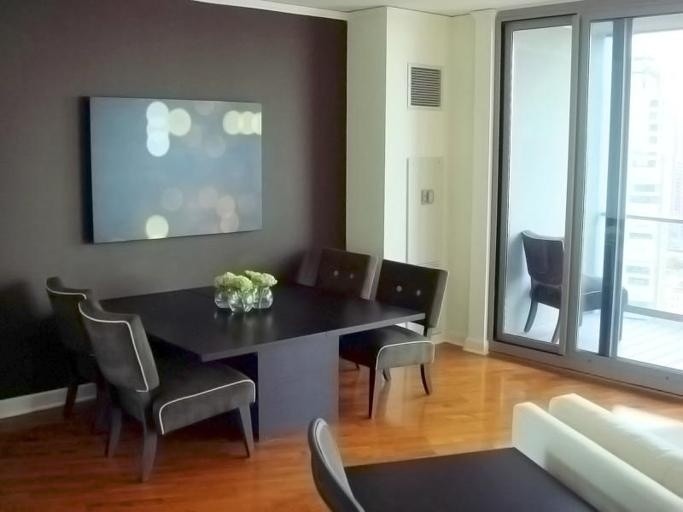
307,417 -> 364,511
522,229 -> 628,344
339,260 -> 448,419
316,247 -> 379,300
44,275 -> 111,435
77,298 -> 257,482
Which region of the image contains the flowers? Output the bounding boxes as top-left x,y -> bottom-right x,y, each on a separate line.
231,275 -> 253,311
213,272 -> 237,301
243,270 -> 278,308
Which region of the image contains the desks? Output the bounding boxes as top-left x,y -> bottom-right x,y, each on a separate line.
343,448 -> 601,511
99,279 -> 426,442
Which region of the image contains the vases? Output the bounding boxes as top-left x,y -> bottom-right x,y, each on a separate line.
214,288 -> 238,308
227,289 -> 255,312
252,286 -> 274,310
